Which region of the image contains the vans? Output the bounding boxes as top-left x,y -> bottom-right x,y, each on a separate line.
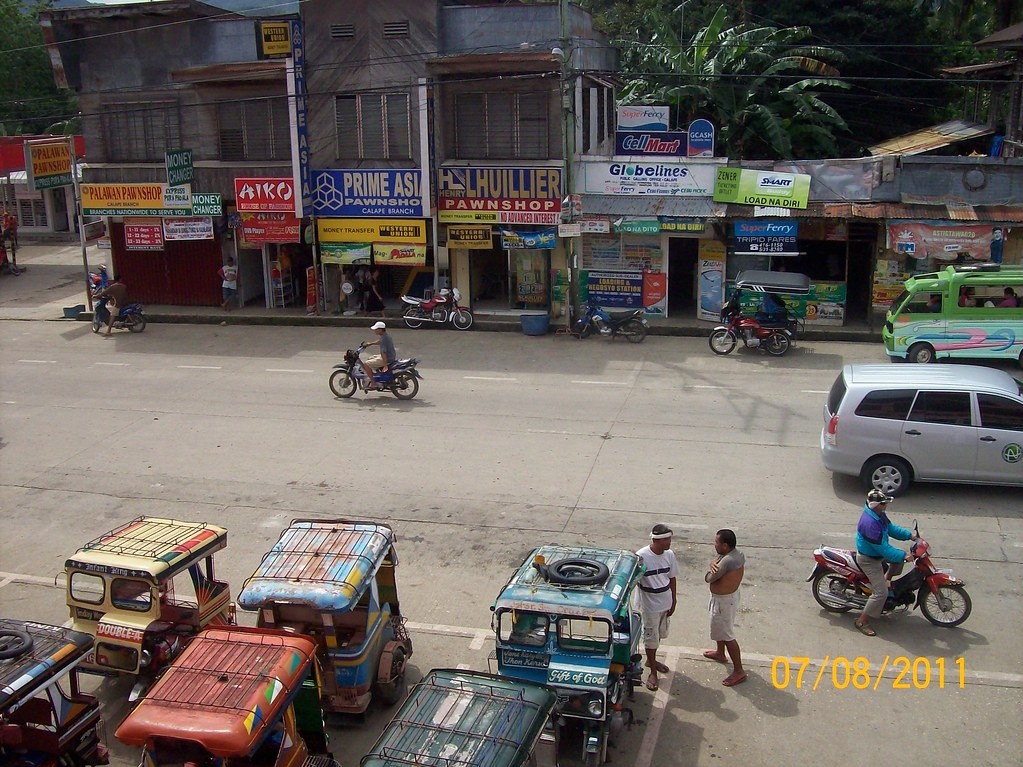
882,263 -> 1023,364
819,363 -> 1022,500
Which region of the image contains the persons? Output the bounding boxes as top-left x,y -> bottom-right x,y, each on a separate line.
634,524 -> 678,690
218,257 -> 237,311
997,287 -> 1018,307
357,266 -> 386,318
704,529 -> 748,686
93,275 -> 129,336
990,228 -> 1003,263
927,296 -> 940,312
854,489 -> 917,636
959,287 -> 975,306
362,322 -> 395,388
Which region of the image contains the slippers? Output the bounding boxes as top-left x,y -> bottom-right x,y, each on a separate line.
722,673 -> 747,686
646,673 -> 658,690
704,650 -> 728,662
645,662 -> 669,672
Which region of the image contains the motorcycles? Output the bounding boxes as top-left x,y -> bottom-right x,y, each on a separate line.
358,669 -> 566,767
114,623 -> 341,767
92,296 -> 149,333
0,619 -> 110,767
401,280 -> 474,331
805,519 -> 973,628
573,295 -> 647,344
234,517 -> 414,726
329,340 -> 424,401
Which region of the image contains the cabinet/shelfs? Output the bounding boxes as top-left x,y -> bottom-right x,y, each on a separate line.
271,261 -> 294,309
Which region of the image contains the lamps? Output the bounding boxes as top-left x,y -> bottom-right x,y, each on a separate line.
552,48 -> 565,63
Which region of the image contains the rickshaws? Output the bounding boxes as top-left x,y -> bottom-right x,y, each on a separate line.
53,513 -> 238,707
709,270 -> 810,357
490,545 -> 648,767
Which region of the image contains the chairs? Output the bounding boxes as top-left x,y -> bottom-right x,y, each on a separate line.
985,301 -> 995,307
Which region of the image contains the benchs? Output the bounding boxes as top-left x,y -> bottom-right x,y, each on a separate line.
509,613 -> 547,645
347,633 -> 365,650
4,697 -> 60,754
274,620 -> 309,633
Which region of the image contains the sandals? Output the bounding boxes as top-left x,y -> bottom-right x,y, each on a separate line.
855,618 -> 875,636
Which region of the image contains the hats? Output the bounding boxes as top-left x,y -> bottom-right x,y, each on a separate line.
867,489 -> 894,501
371,321 -> 385,330
439,288 -> 449,295
